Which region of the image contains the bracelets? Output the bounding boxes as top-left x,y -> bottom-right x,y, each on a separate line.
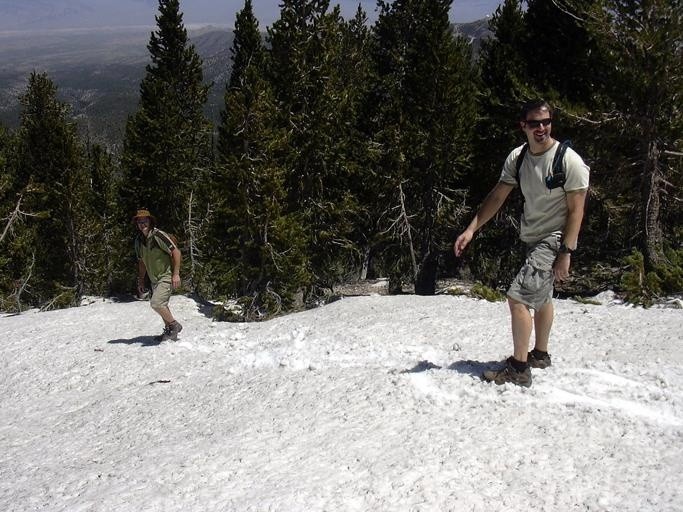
137,277 -> 144,285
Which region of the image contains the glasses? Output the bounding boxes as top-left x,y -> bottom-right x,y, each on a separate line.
527,118 -> 551,128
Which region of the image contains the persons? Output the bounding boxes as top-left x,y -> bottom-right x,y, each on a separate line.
454,98 -> 591,386
132,210 -> 183,341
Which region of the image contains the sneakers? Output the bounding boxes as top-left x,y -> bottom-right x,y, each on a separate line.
483,356 -> 532,388
154,320 -> 183,344
527,347 -> 551,368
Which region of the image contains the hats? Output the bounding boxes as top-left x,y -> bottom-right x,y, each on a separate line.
130,210 -> 156,226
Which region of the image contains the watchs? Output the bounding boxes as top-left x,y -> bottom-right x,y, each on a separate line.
559,244 -> 574,254
